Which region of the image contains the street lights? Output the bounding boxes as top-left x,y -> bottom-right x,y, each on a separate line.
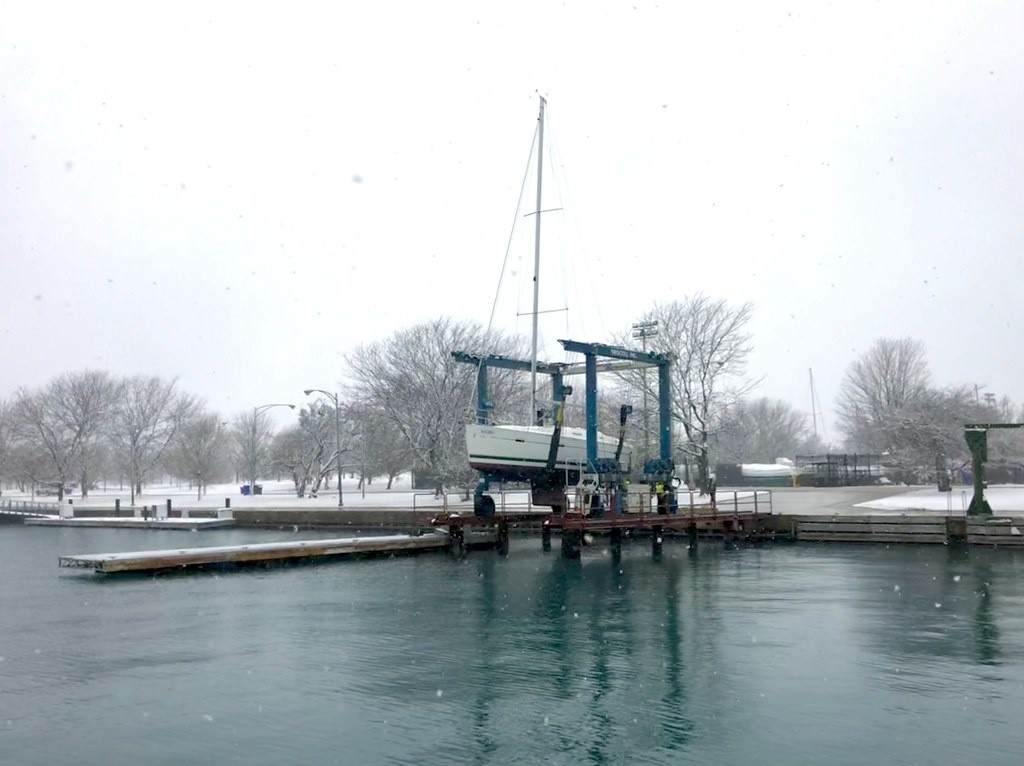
248,403 -> 295,496
303,388 -> 343,506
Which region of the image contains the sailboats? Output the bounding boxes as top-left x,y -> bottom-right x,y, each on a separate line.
465,86 -> 634,477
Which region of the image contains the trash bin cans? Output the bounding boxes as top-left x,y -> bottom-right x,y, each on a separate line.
255,484 -> 262,494
241,485 -> 249,495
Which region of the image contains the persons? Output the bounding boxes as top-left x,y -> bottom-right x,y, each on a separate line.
708,479 -> 718,509
654,481 -> 673,514
